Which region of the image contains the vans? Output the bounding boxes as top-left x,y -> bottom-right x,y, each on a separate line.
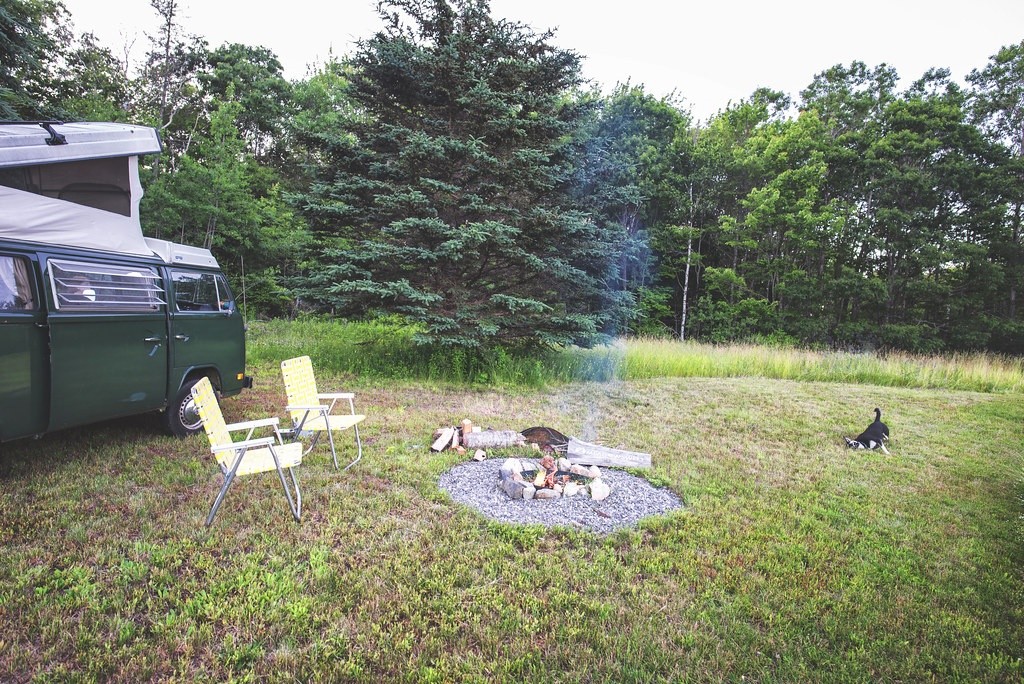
0,118 -> 254,443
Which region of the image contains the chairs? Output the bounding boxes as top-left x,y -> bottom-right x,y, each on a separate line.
282,357 -> 361,471
190,376 -> 304,527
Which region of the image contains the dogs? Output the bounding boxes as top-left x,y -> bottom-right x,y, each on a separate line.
843,407 -> 892,457
